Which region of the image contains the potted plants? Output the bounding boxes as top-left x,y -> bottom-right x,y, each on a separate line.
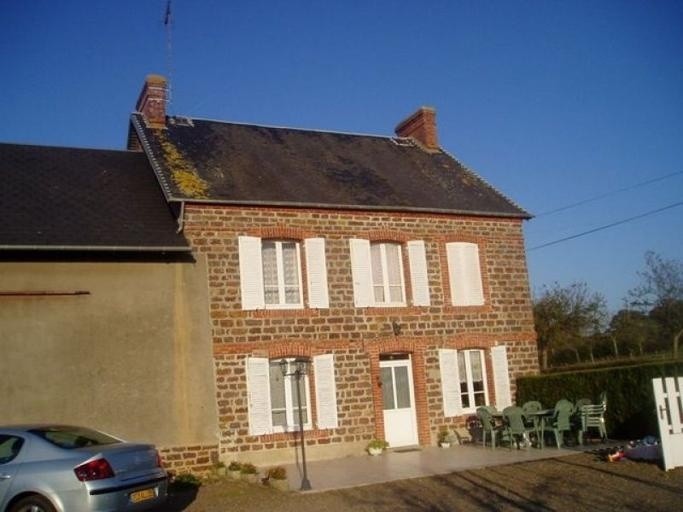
366,440 -> 390,456
437,430 -> 451,448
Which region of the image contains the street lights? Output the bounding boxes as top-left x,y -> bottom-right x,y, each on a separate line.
276,351 -> 316,491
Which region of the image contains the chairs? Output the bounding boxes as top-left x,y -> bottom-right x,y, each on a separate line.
477,391 -> 608,448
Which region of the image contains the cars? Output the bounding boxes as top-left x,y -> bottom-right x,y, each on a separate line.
0,421 -> 171,512
